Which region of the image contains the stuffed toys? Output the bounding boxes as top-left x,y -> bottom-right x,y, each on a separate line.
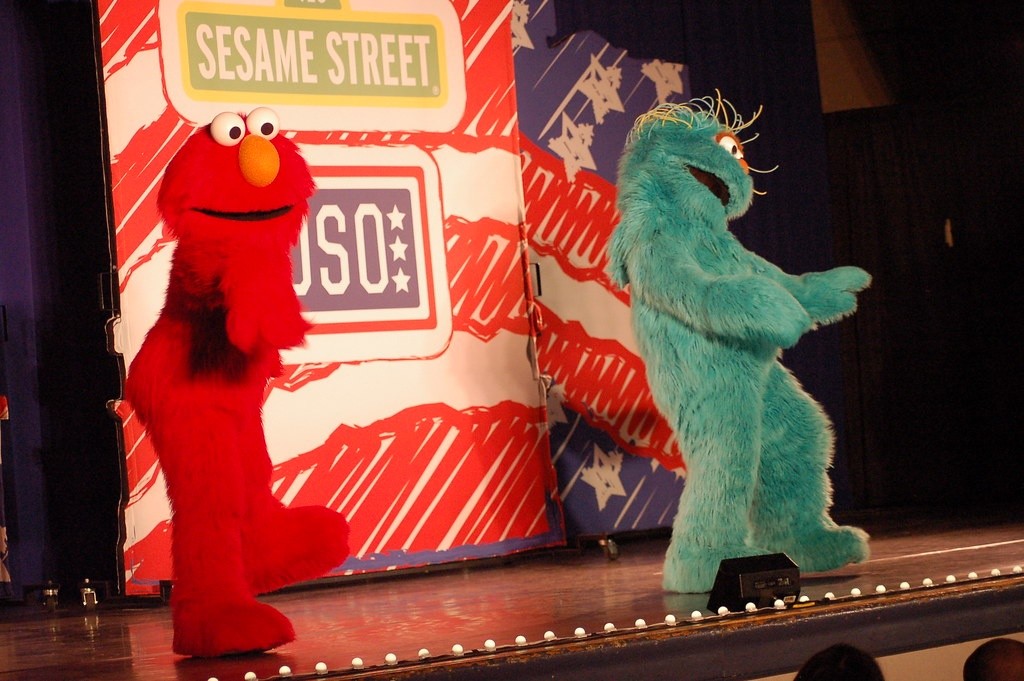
603,88 -> 874,600
122,107 -> 352,660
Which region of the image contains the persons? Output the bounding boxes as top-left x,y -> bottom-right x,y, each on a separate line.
961,637 -> 1024,681
792,642 -> 884,681
